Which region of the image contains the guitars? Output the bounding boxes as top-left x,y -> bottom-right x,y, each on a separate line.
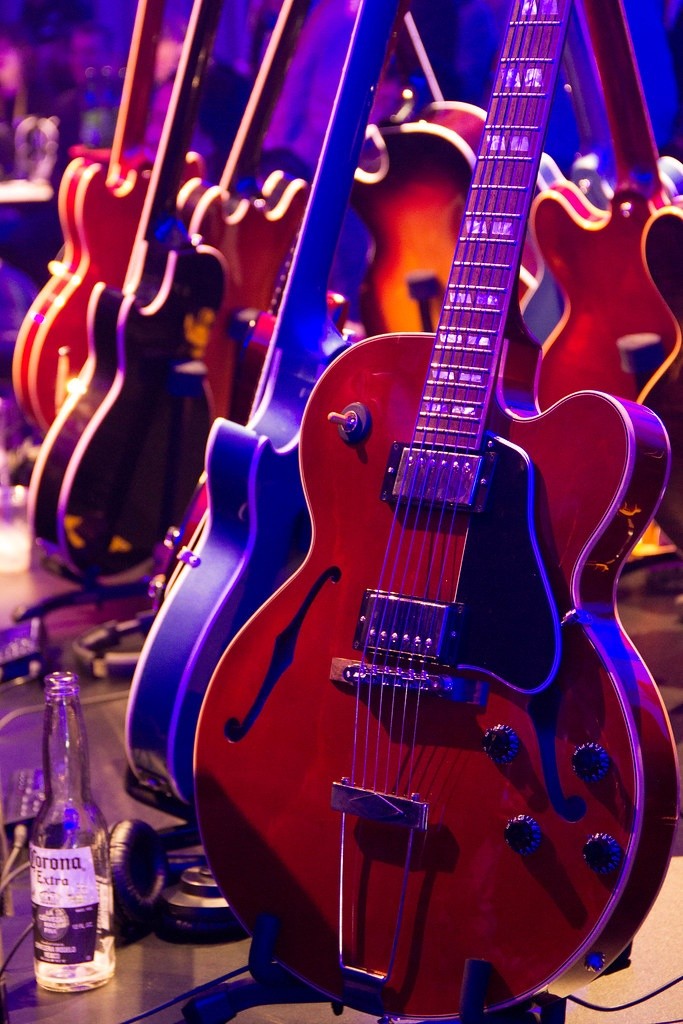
190,2 -> 682,1021
15,2 -> 681,594
126,2 -> 401,821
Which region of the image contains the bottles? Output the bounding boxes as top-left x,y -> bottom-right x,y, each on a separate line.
26,670 -> 117,991
